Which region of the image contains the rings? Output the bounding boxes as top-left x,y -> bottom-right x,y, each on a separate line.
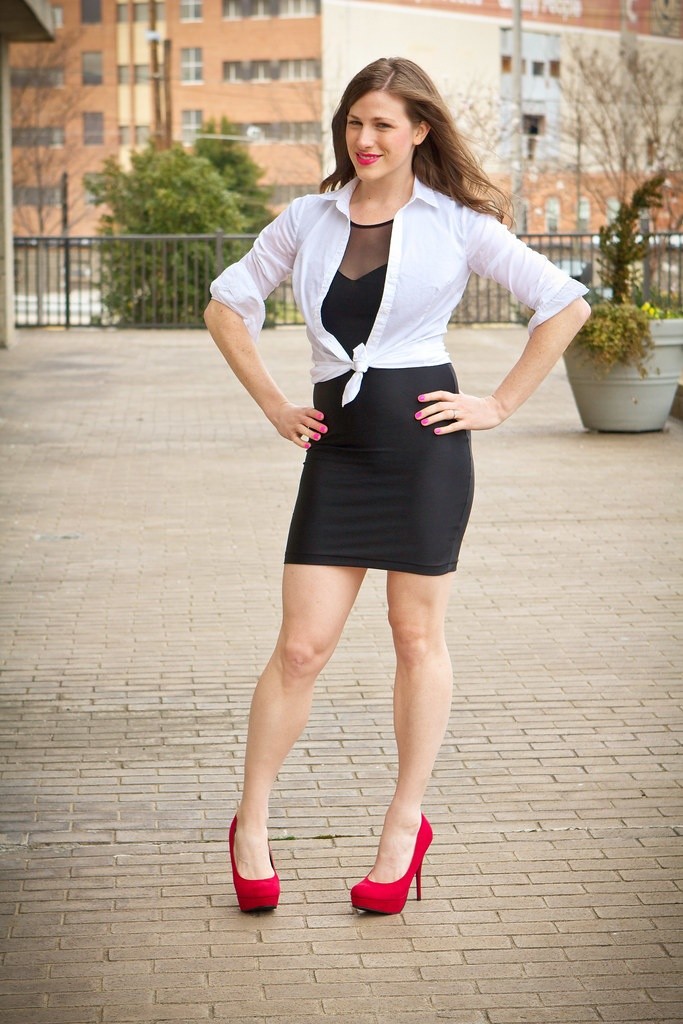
453,410 -> 455,419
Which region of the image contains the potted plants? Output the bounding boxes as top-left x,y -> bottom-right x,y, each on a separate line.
560,169 -> 683,432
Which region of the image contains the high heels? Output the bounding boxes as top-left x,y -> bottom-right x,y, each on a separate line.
226,811 -> 280,913
348,812 -> 434,914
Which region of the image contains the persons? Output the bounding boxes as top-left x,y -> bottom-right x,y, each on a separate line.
205,57 -> 591,915
